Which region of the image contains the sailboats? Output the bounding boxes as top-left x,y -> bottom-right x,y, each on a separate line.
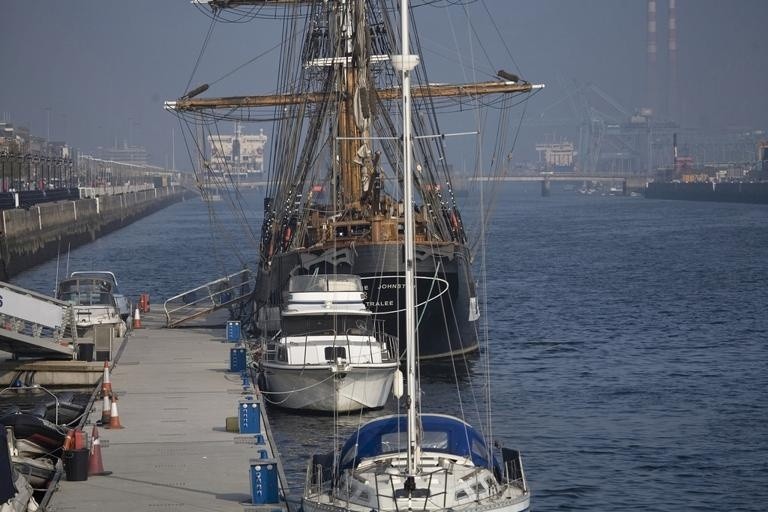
301,0 -> 530,512
164,1 -> 544,369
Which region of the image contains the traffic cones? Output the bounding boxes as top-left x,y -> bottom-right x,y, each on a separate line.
134,304 -> 142,329
101,360 -> 116,399
100,389 -> 111,423
106,392 -> 123,429
86,426 -> 111,476
140,293 -> 144,311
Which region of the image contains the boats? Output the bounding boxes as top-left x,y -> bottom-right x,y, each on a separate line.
260,275 -> 405,412
58,269 -> 133,318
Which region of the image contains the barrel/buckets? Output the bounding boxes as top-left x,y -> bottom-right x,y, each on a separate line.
63,449 -> 89,482
96,351 -> 109,361
78,343 -> 94,361
